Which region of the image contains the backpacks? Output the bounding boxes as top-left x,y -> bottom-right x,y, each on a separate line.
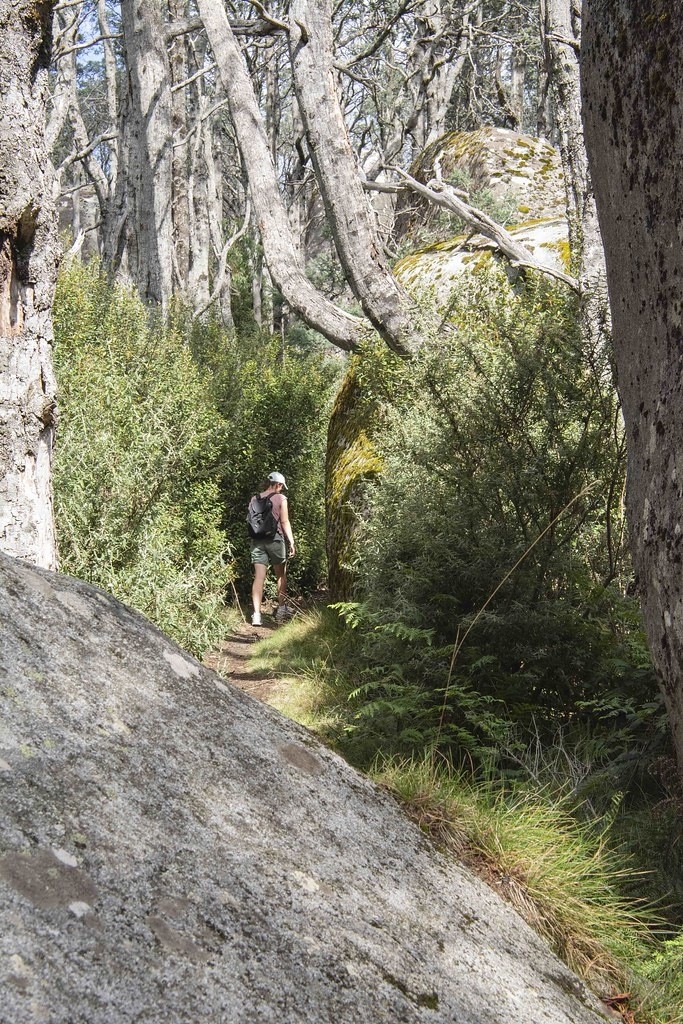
245,492 -> 279,540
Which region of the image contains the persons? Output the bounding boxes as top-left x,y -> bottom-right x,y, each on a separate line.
246,472 -> 295,625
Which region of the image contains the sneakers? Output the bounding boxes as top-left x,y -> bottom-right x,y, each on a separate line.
251,612 -> 263,626
276,604 -> 294,620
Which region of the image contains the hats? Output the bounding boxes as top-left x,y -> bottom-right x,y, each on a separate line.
268,471 -> 288,490
